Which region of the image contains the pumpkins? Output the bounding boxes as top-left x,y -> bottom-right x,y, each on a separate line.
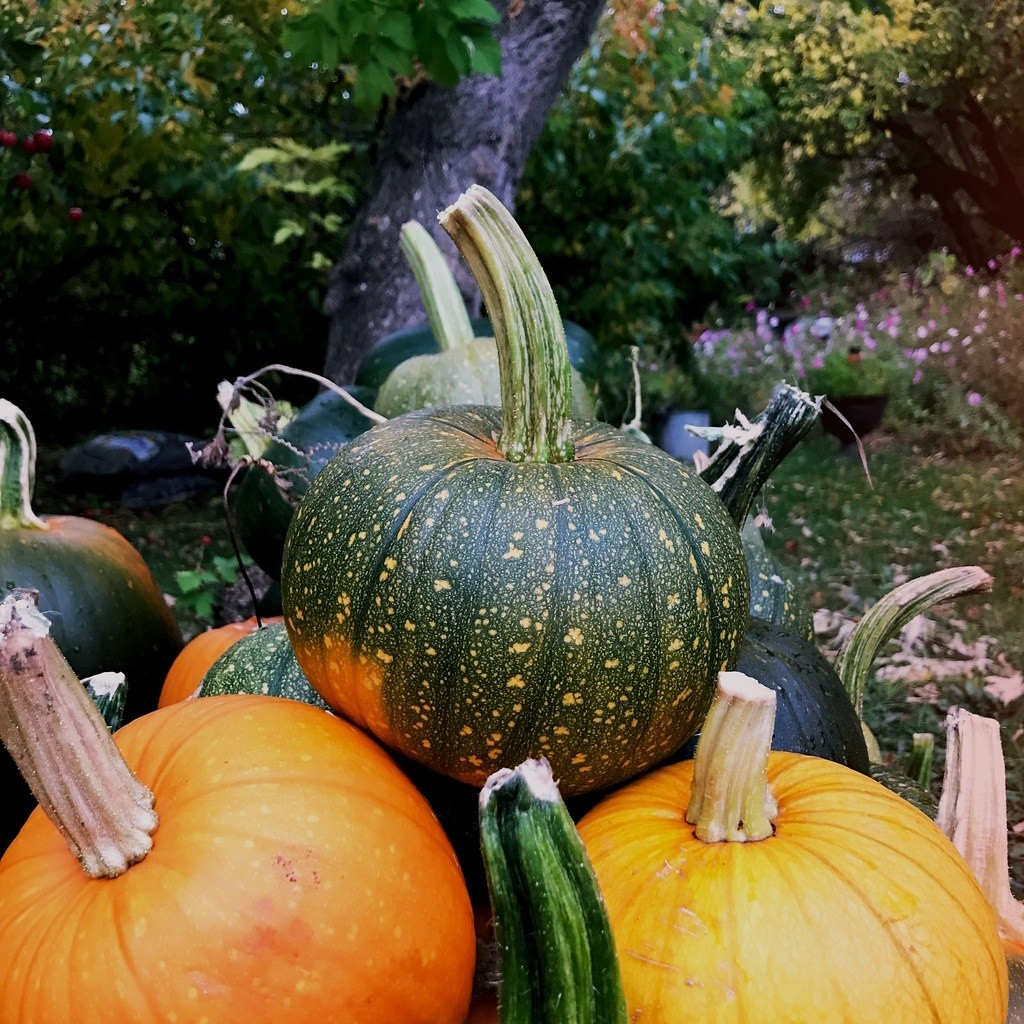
0,185 -> 1024,1024
